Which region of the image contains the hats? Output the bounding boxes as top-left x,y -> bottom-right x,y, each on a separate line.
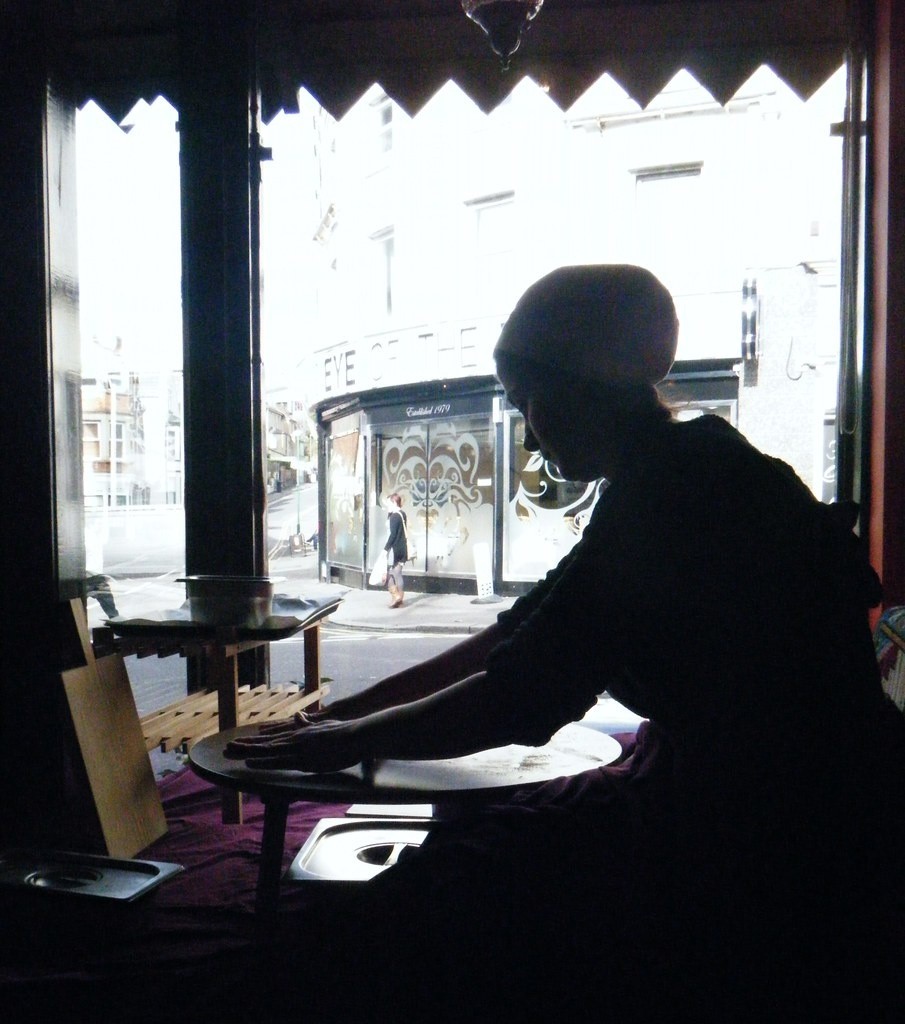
493,264 -> 679,387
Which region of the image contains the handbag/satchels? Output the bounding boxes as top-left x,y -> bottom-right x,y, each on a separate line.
406,538 -> 411,560
369,552 -> 388,586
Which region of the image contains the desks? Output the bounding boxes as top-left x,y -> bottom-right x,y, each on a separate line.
192,719 -> 624,925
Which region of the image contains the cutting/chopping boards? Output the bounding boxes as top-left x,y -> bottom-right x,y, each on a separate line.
60,597 -> 167,860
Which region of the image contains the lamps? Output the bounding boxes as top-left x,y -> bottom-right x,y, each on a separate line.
460,0 -> 545,72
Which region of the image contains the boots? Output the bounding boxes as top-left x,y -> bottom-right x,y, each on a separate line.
388,585 -> 404,607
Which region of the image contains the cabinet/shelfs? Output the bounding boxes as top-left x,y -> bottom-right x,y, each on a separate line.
110,590 -> 343,821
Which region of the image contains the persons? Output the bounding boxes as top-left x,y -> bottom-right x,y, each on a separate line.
380,493 -> 409,608
222,264 -> 905,1024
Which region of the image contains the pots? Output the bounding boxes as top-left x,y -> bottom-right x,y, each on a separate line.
174,571 -> 286,627
